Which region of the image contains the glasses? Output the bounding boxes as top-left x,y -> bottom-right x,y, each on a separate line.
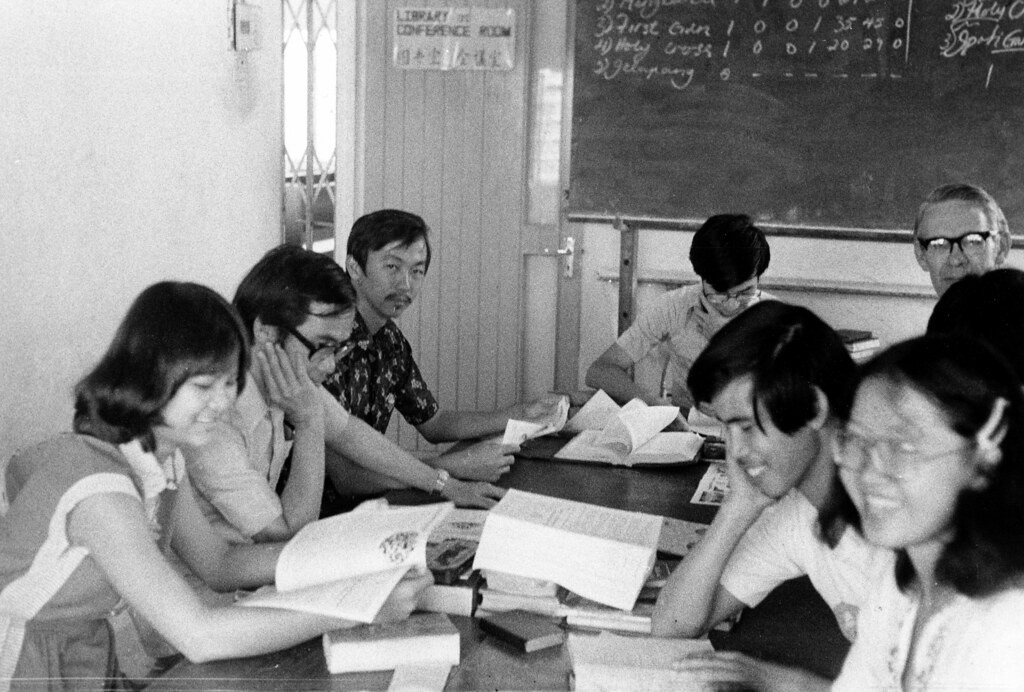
286,325 -> 358,363
702,277 -> 762,304
828,421 -> 978,480
917,230 -> 1000,258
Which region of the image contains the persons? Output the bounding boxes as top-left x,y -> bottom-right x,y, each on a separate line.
178,243 -> 507,546
0,280 -> 435,690
828,183 -> 1023,692
652,301 -> 895,645
274,210 -> 563,519
586,213 -> 779,431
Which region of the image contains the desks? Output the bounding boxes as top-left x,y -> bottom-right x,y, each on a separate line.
144,405 -> 850,692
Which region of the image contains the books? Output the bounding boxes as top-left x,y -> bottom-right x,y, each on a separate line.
833,329 -> 878,363
235,486 -> 744,675
501,396 -> 570,447
554,398 -> 706,466
567,633 -> 716,691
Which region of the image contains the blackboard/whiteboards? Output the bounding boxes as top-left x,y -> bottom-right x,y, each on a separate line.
556,1 -> 1024,252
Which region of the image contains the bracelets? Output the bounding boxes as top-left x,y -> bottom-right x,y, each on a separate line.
431,467 -> 449,502
